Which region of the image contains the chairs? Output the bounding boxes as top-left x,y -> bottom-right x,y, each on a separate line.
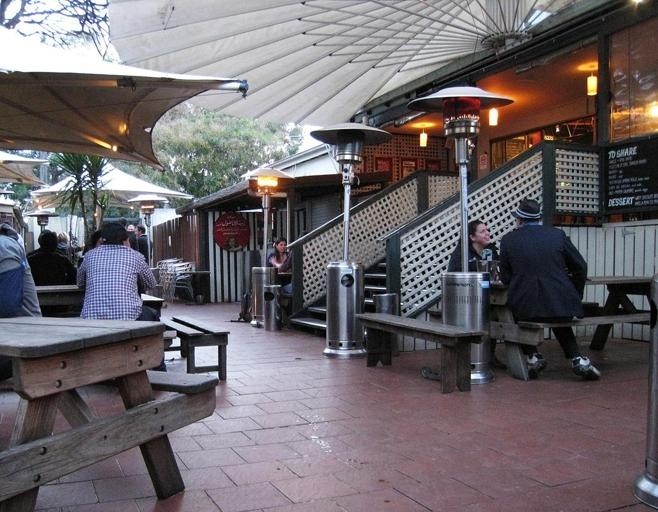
156,257 -> 195,304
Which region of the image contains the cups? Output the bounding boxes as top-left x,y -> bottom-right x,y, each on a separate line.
476,260 -> 489,273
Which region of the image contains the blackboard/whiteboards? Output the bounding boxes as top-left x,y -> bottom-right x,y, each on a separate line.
607,134 -> 658,215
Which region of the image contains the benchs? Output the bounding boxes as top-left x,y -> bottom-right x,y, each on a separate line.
98,367 -> 220,396
139,293 -> 165,319
427,300 -> 599,324
355,312 -> 483,393
517,312 -> 653,346
161,312 -> 232,382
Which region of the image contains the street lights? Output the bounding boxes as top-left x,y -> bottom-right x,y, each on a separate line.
129,192 -> 167,271
408,84 -> 513,387
310,121 -> 394,362
240,164 -> 296,328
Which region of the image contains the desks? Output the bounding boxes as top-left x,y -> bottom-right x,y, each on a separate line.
0,285 -> 166,324
484,275 -> 658,380
0,316 -> 184,512
180,270 -> 212,304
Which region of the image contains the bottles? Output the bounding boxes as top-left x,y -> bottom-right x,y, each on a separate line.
491,260 -> 501,282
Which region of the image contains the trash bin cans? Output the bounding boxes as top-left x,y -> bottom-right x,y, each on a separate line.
262,284 -> 283,331
372,293 -> 399,358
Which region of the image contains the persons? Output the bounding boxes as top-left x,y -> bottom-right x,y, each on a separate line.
221,235 -> 244,252
446,221 -> 498,273
269,231 -> 312,294
1,222 -> 45,318
79,217 -> 152,267
266,237 -> 289,267
56,232 -> 73,249
76,221 -> 167,369
28,230 -> 75,285
499,196 -> 602,382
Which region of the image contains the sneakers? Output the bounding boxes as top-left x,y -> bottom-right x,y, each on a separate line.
571,356 -> 601,378
528,353 -> 547,375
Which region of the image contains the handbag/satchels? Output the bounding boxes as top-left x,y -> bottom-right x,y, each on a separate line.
0,267 -> 24,318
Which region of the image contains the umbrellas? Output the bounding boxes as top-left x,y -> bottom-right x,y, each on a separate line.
29,157 -> 195,229
0,150 -> 49,186
107,0 -> 575,125
0,23 -> 248,172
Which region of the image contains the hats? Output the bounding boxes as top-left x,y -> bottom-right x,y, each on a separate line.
511,199 -> 543,222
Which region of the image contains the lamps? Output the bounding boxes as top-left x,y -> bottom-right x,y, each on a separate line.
420,125 -> 428,147
488,107 -> 499,126
586,65 -> 597,96
